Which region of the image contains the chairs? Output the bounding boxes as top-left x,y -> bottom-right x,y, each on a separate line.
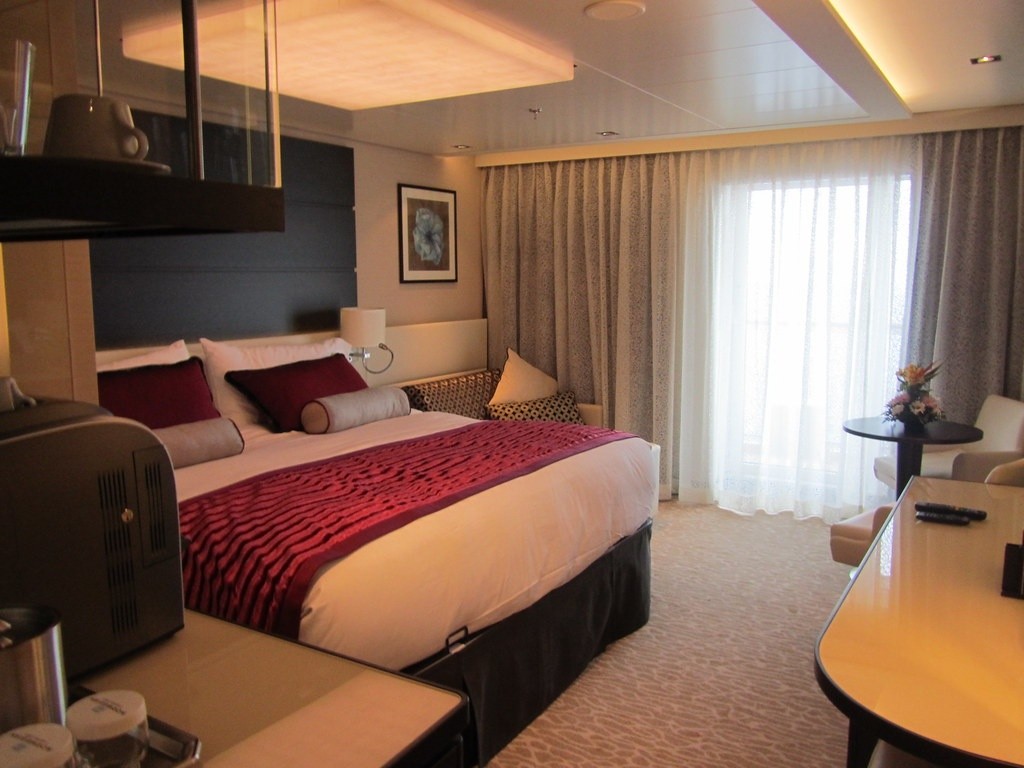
873,395 -> 1024,489
831,457 -> 1024,567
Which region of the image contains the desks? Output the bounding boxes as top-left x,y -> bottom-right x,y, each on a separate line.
845,415 -> 984,501
808,474 -> 1024,768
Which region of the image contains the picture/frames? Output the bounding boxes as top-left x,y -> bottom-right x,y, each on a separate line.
398,183 -> 461,283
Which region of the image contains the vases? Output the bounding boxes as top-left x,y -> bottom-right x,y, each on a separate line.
898,404 -> 932,428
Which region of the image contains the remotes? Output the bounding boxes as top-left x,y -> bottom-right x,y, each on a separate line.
914,512 -> 970,525
915,502 -> 986,520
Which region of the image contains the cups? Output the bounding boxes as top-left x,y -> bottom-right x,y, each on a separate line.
0,607 -> 68,729
0,36 -> 37,156
42,94 -> 150,159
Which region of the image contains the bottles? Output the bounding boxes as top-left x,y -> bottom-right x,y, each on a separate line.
0,721 -> 79,768
63,689 -> 151,768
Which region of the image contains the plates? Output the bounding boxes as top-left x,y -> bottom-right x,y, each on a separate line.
51,152 -> 171,177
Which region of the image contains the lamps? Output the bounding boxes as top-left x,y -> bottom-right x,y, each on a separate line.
340,307 -> 394,374
122,0 -> 575,110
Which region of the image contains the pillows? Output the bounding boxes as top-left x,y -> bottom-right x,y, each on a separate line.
488,346 -> 560,402
404,369 -> 502,421
226,353 -> 370,432
486,392 -> 585,428
97,357 -> 214,430
202,337 -> 350,424
148,419 -> 245,470
299,389 -> 412,435
96,339 -> 189,373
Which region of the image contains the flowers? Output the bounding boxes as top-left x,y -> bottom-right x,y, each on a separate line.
882,357 -> 945,419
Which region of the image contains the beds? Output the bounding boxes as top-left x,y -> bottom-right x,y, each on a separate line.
96,331 -> 671,767
